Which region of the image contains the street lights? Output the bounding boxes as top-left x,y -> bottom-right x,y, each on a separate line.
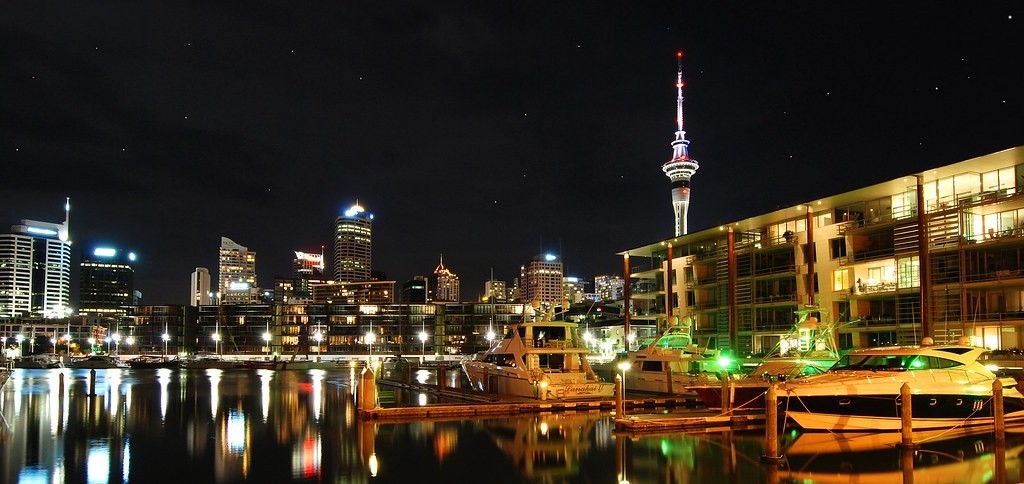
52,330 -> 58,354
90,333 -> 96,355
214,320 -> 221,354
64,322 -> 72,356
419,321 -> 428,357
366,319 -> 376,356
486,318 -> 496,348
263,320 -> 272,356
164,322 -> 169,355
315,320 -> 322,358
106,322 -> 135,355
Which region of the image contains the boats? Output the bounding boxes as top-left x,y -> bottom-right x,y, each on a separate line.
746,298 -> 1024,455
459,295 -> 615,400
124,355 -> 234,369
596,324 -> 739,394
12,355 -> 129,369
